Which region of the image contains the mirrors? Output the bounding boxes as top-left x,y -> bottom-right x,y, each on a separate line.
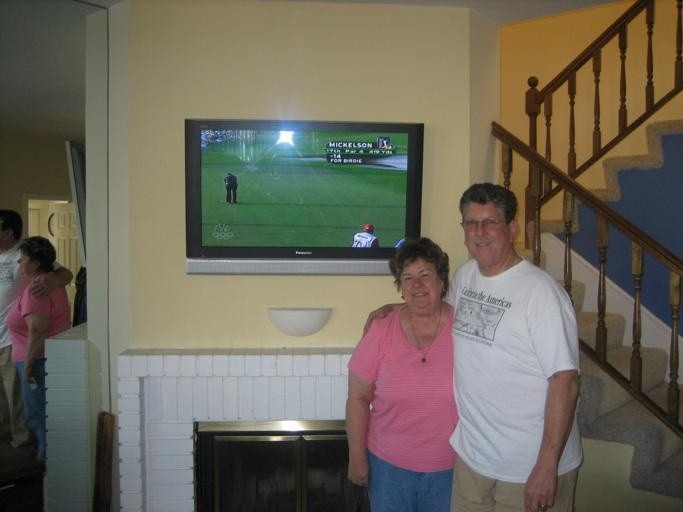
1,0 -> 110,512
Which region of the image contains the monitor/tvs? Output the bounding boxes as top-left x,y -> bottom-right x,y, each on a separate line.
63,137 -> 86,257
184,119 -> 424,273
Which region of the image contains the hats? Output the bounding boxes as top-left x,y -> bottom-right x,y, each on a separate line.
364,224 -> 374,232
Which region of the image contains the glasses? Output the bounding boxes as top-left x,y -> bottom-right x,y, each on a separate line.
460,217 -> 505,229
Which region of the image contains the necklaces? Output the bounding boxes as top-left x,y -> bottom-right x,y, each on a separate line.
407,304 -> 443,365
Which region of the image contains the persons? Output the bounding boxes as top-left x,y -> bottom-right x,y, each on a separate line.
7,236 -> 70,472
362,183 -> 583,512
353,224 -> 378,249
224,173 -> 237,203
0,209 -> 73,449
345,236 -> 459,511
